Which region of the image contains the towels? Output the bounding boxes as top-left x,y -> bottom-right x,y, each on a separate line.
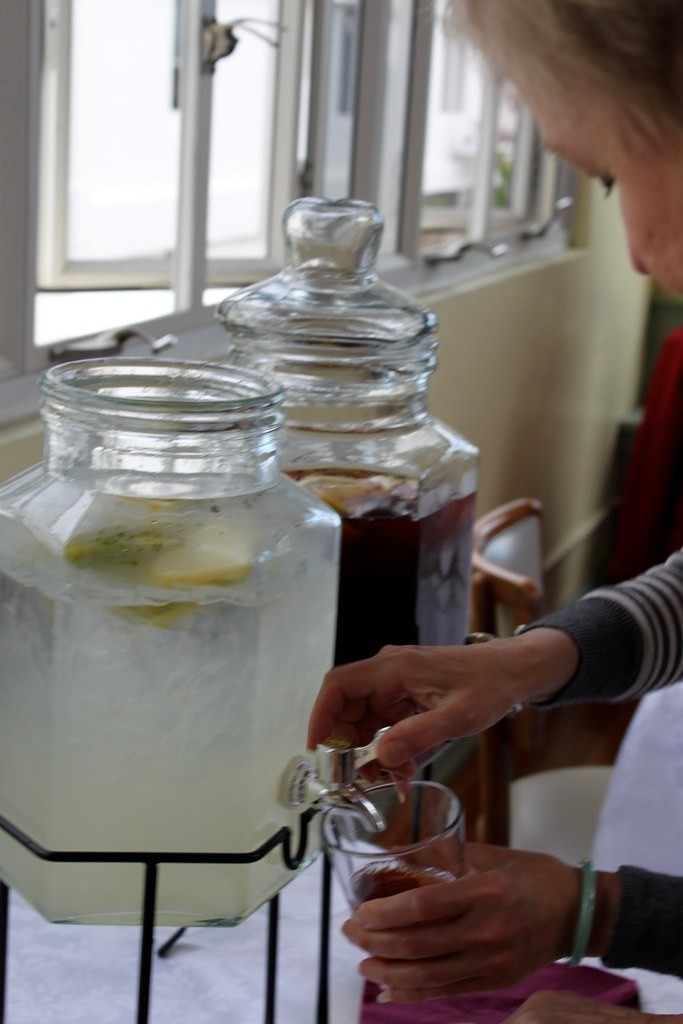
360,962 -> 642,1024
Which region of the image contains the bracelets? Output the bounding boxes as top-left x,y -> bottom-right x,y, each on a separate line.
566,859 -> 596,968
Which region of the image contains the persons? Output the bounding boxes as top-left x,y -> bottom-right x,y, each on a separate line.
307,2 -> 682,1024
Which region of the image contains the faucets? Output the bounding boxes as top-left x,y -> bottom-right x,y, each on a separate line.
291,740 -> 387,836
463,624 -> 533,716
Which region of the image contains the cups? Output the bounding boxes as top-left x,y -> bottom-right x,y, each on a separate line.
319,778 -> 466,911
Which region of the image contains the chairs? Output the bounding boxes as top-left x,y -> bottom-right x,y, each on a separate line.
472,489 -> 639,867
